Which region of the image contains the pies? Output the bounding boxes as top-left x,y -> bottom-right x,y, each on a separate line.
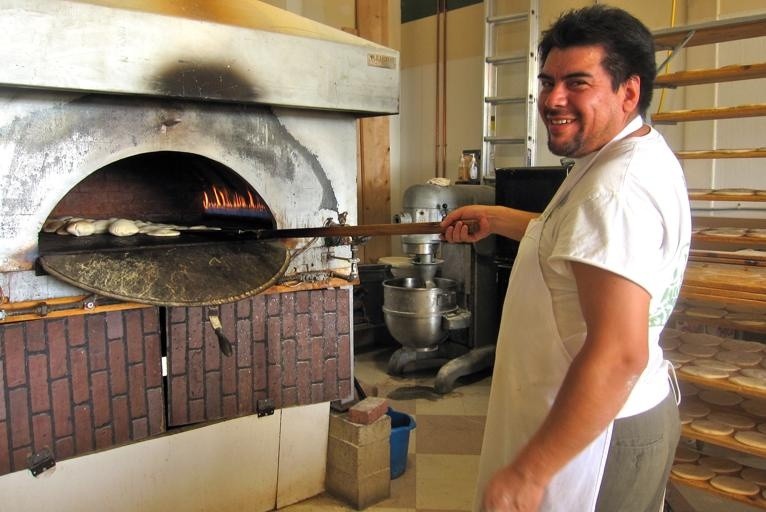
43,215 -> 221,236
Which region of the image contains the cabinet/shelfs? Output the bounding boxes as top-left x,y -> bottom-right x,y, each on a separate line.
657,263 -> 766,511
647,18 -> 765,259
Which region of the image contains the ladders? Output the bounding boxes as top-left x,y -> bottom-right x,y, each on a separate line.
481,0 -> 539,184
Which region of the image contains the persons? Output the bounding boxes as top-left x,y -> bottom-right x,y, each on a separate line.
439,4 -> 692,511
